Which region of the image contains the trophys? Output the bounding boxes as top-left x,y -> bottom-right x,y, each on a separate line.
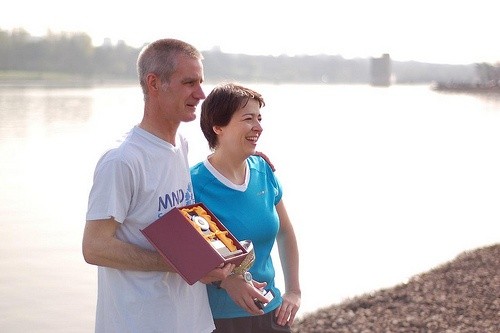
231,240 -> 274,310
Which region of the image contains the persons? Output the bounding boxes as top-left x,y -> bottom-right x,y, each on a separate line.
81,38 -> 275,333
190,83 -> 301,333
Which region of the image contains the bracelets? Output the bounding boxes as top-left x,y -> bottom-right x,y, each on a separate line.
211,280 -> 222,287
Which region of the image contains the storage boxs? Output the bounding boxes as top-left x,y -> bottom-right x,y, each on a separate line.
138,203 -> 249,285
